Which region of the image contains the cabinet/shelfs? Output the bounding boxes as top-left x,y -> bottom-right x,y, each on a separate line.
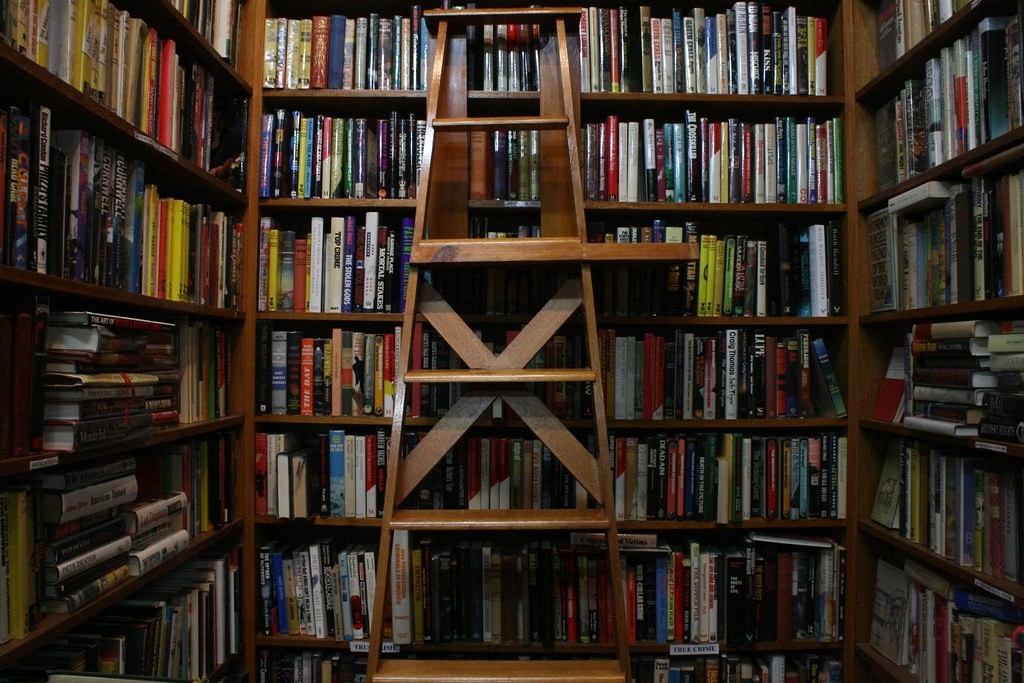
0,0 -> 1024,683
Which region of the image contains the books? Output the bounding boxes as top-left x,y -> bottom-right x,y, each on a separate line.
0,1 -> 1024,683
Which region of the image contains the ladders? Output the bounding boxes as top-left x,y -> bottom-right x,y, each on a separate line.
354,0 -> 658,683
